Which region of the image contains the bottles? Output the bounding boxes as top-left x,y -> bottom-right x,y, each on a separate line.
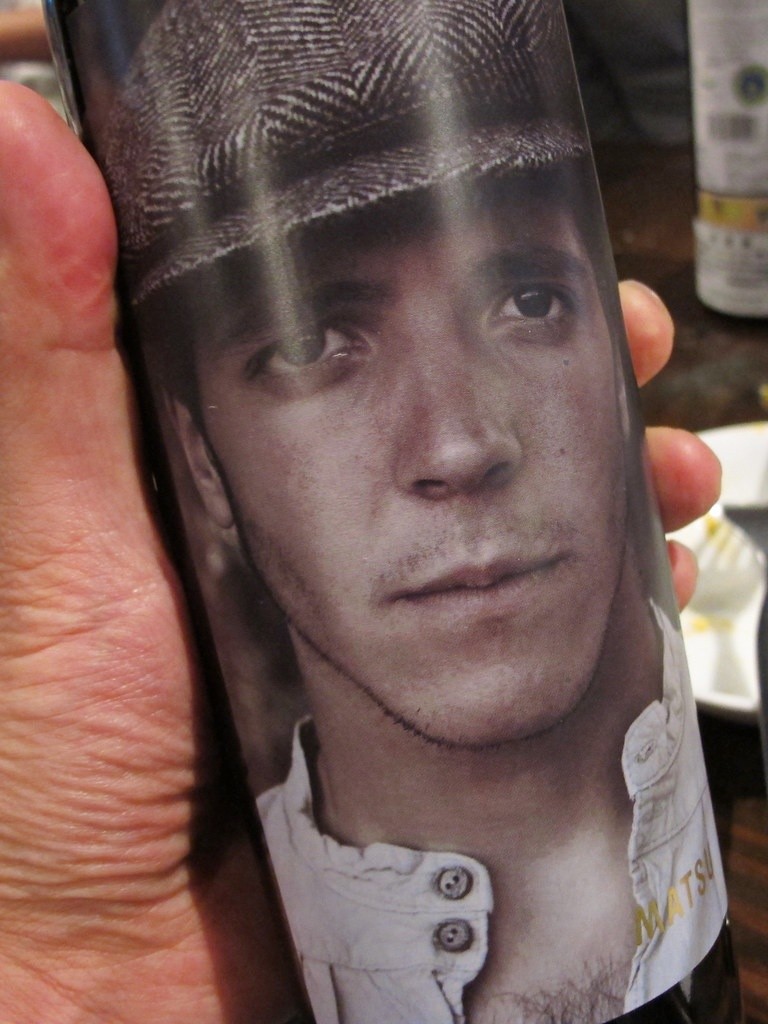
45,0 -> 742,1024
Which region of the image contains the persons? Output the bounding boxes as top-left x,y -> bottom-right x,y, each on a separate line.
106,0 -> 746,1024
0,78 -> 725,1024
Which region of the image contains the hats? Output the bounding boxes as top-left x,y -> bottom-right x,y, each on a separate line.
68,0 -> 589,309
